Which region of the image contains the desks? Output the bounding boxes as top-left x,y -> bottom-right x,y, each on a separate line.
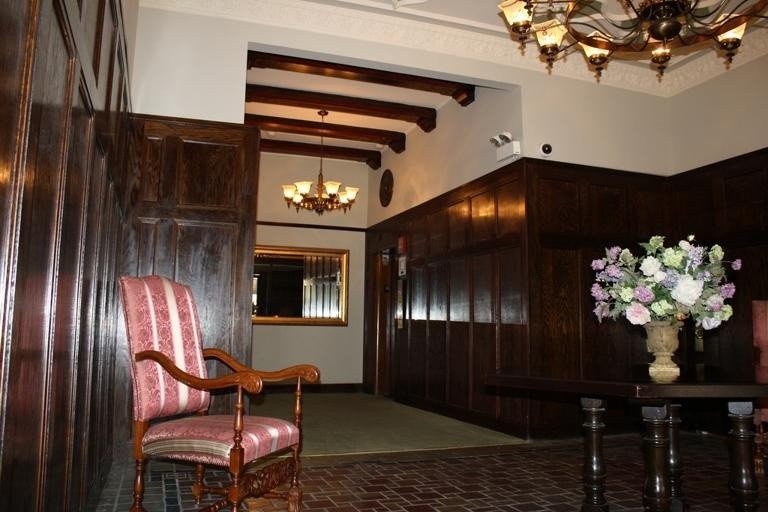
476,363 -> 766,512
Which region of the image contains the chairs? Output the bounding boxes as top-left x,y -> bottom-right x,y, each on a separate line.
119,267 -> 322,510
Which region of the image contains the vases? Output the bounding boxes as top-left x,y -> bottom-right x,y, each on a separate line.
639,314 -> 684,384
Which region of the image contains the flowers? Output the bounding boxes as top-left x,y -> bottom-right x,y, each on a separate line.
580,214 -> 741,328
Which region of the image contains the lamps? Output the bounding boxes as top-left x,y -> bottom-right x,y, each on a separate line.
283,113 -> 361,216
489,132 -> 519,162
499,0 -> 767,80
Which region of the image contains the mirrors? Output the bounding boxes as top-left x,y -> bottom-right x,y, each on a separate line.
252,243 -> 349,327
